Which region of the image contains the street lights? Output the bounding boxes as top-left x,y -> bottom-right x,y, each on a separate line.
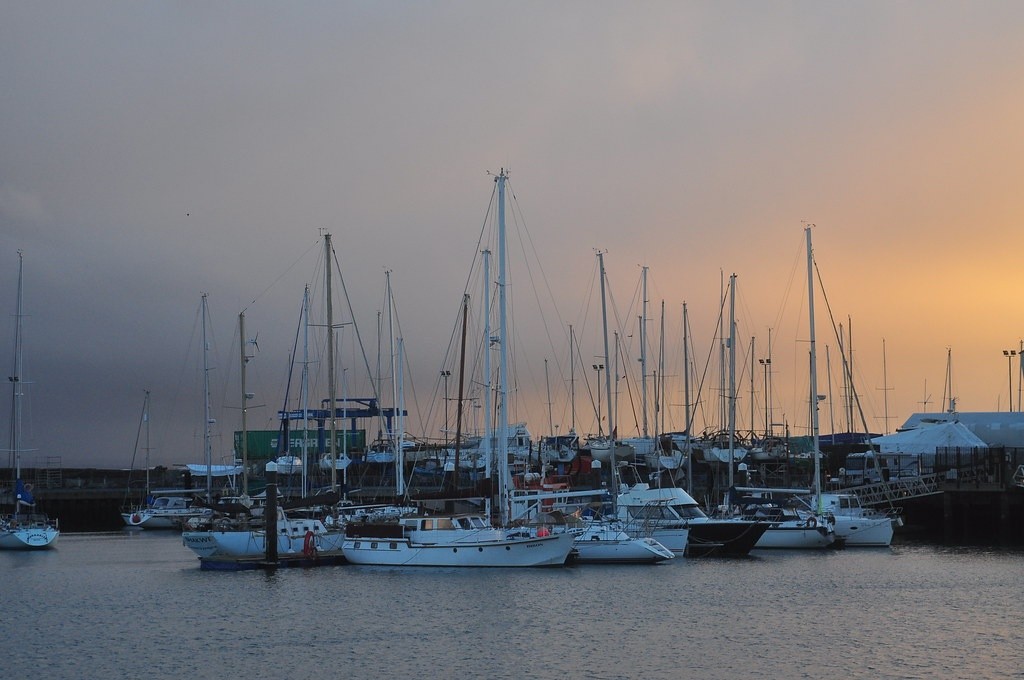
440,370 -> 452,445
8,375 -> 20,482
1003,348 -> 1016,414
591,364 -> 605,439
759,359 -> 773,440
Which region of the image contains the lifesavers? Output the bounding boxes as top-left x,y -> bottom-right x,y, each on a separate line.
219,517 -> 231,528
806,517 -> 817,527
303,531 -> 316,560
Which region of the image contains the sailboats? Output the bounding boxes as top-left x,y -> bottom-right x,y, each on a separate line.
123,164 -> 1024,566
0,247 -> 60,549
